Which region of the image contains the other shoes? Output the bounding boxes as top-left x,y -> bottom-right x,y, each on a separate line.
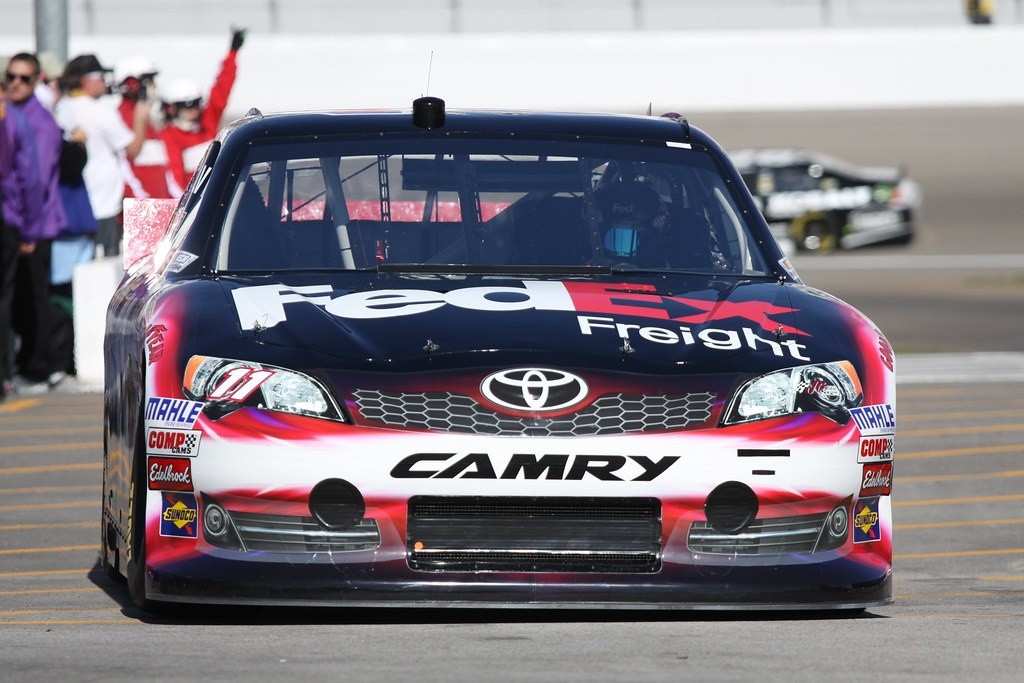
18,372 -> 78,397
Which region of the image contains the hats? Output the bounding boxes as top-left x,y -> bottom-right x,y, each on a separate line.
64,55 -> 113,86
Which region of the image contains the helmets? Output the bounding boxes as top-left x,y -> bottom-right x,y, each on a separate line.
159,78 -> 201,119
115,54 -> 158,98
578,179 -> 675,266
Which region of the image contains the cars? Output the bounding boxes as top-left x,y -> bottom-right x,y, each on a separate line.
102,96 -> 900,611
729,145 -> 919,257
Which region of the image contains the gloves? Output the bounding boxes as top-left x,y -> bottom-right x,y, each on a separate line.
231,28 -> 245,52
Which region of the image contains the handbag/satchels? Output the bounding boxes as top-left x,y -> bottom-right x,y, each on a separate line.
58,140 -> 87,188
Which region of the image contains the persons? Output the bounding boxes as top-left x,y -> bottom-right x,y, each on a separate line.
576,180 -> 672,267
0,26 -> 250,401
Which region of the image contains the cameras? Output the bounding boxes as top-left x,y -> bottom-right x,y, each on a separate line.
137,72 -> 158,100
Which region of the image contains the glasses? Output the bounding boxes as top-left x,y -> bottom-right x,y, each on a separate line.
7,74 -> 35,84
602,226 -> 644,259
